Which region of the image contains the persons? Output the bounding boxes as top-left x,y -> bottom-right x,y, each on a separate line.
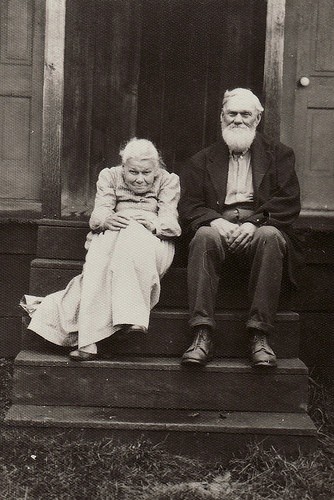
181,88 -> 301,368
68,137 -> 182,361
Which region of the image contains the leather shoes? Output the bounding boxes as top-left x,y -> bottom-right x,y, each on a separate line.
180,326 -> 214,367
244,329 -> 277,368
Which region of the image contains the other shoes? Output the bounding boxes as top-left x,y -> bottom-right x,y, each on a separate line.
127,325 -> 147,334
68,348 -> 96,360
